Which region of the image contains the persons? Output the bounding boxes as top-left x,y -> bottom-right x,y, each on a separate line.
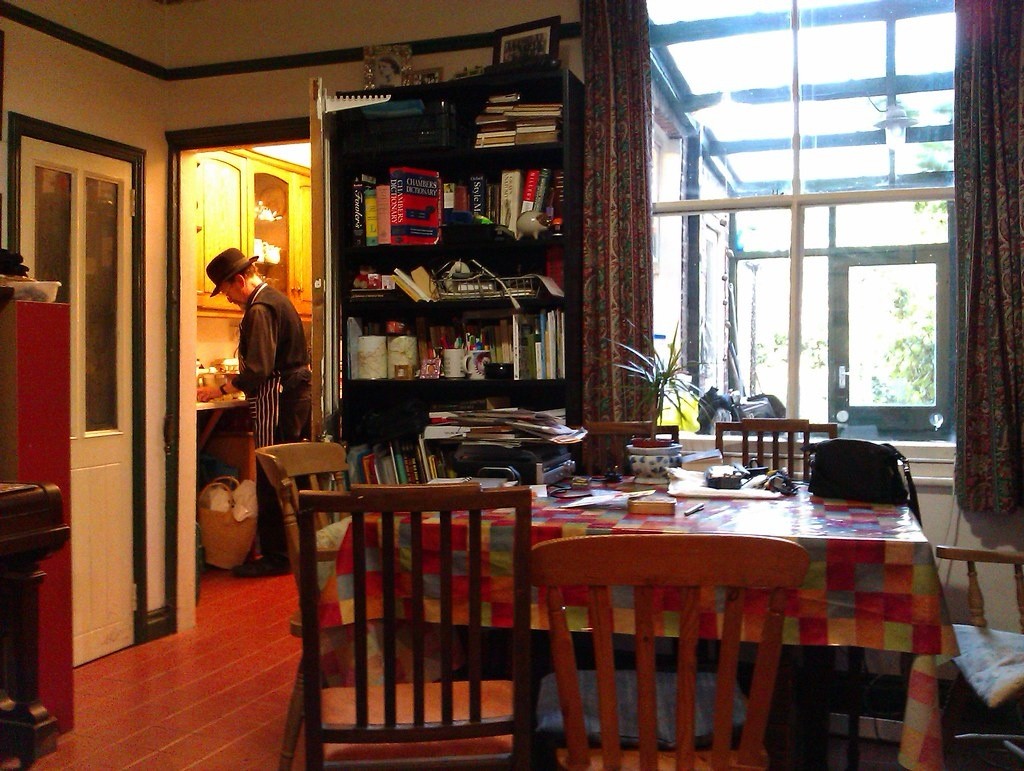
196,247 -> 311,578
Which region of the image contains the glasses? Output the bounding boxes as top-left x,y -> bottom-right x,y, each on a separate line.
220,283 -> 231,296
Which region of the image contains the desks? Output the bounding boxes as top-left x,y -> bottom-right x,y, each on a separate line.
360,472 -> 930,771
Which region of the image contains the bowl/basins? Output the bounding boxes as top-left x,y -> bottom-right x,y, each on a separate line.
8,282 -> 62,302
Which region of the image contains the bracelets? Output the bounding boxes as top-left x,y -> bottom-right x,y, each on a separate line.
220,383 -> 228,395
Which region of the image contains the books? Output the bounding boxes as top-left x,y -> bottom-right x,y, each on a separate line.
475,92 -> 562,148
390,264 -> 537,304
682,448 -> 723,471
341,398 -> 588,488
340,164 -> 563,248
345,308 -> 564,381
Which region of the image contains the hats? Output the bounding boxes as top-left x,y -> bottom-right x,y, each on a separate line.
206,247 -> 259,297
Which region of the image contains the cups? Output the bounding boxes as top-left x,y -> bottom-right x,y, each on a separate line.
444,349 -> 465,378
464,350 -> 491,380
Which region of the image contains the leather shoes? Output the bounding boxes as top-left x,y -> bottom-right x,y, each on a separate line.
231,551 -> 290,578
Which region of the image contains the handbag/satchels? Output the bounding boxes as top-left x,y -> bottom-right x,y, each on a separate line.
799,439 -> 922,528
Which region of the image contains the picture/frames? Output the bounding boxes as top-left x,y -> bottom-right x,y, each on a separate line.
492,15 -> 561,66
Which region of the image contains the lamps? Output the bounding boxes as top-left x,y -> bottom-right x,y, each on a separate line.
866,90 -> 918,152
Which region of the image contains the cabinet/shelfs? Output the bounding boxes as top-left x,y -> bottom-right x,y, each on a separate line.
248,150 -> 311,322
197,147 -> 249,319
323,58 -> 587,492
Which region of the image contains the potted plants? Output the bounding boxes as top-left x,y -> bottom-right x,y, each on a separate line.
591,314 -> 716,486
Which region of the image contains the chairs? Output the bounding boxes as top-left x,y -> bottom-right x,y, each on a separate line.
295,481 -> 533,771
935,544 -> 1024,771
563,421 -> 681,475
529,531 -> 811,771
715,418 -> 839,482
255,440 -> 352,771
